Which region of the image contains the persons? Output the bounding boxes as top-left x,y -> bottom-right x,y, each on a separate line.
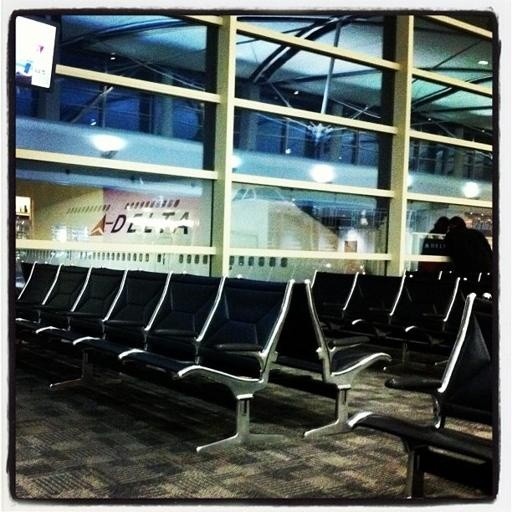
419,216 -> 450,271
445,216 -> 492,277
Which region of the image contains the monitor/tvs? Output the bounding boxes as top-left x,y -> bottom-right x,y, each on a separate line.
15,193 -> 31,216
14,15 -> 62,94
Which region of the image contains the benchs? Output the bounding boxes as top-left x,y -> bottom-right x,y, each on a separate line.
309,261 -> 493,497
18,260 -> 409,457
